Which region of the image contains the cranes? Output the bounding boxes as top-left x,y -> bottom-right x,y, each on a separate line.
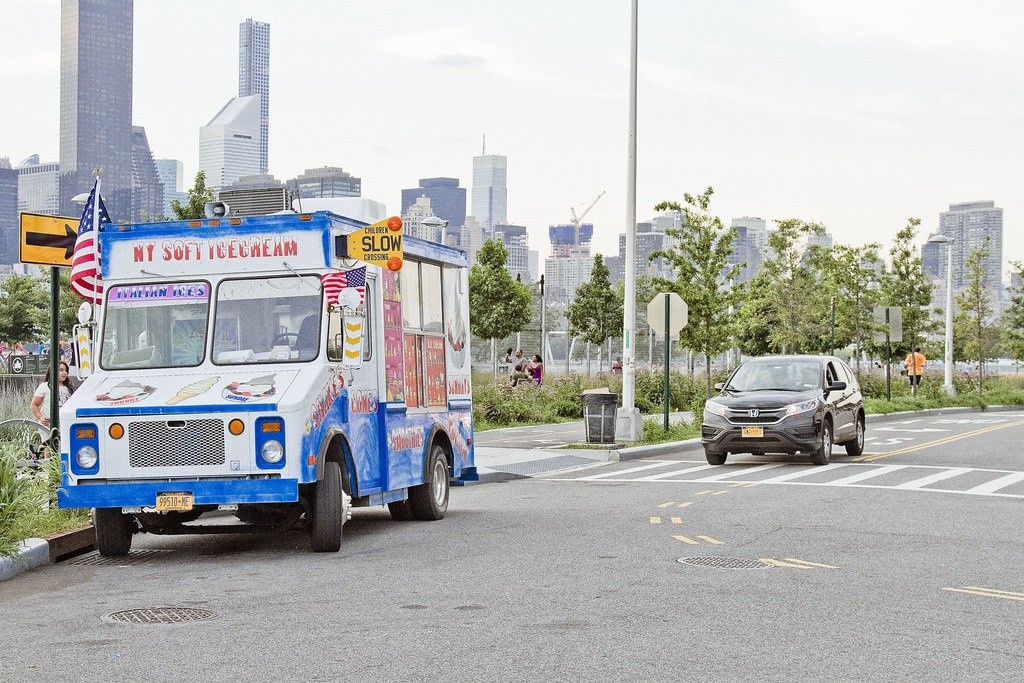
571,190 -> 606,250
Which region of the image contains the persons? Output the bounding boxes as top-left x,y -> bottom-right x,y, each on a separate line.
29,359 -> 75,454
505,349 -> 543,388
28,342 -> 47,355
796,365 -> 828,388
59,335 -> 80,367
903,347 -> 927,394
612,357 -> 623,375
59,345 -> 65,363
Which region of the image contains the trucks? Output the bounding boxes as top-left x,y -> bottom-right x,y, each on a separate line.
58,201 -> 479,558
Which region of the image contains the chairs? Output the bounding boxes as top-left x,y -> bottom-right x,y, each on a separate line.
517,378 -> 527,386
530,378 -> 539,385
295,316 -> 317,351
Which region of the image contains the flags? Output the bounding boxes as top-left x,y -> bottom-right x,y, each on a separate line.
69,181 -> 112,305
321,265 -> 365,303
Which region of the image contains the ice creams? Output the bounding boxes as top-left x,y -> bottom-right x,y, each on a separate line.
222,373 -> 276,403
165,376 -> 220,405
95,379 -> 157,406
447,282 -> 466,369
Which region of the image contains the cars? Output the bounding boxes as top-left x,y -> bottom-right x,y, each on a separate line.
702,354 -> 867,465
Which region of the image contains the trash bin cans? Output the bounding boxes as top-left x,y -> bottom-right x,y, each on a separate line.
581,392 -> 620,444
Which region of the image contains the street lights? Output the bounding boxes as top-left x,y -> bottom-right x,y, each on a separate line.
928,235 -> 958,402
